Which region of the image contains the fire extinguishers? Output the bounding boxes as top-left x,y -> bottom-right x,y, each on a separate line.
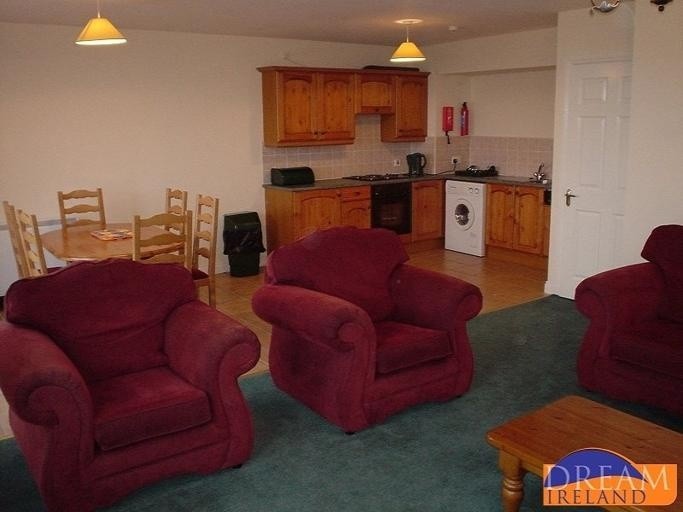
460,102 -> 468,136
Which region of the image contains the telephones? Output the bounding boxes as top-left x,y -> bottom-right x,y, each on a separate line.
442,107 -> 453,131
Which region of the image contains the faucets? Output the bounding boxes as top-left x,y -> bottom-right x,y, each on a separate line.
534,163 -> 545,181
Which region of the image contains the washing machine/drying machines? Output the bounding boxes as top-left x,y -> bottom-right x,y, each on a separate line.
444,180 -> 485,258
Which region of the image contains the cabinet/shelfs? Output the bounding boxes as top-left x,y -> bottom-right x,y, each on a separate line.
355,70 -> 394,114
257,66 -> 356,147
381,71 -> 433,142
266,188 -> 371,257
485,183 -> 543,255
412,180 -> 445,252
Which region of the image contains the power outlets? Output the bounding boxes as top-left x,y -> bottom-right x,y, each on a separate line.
452,156 -> 459,164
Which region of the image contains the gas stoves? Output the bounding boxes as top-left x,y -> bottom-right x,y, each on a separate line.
341,172 -> 409,182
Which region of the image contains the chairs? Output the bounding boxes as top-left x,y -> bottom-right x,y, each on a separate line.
192,193 -> 219,307
0,256 -> 261,512
573,225 -> 683,415
252,225 -> 482,434
133,209 -> 192,272
3,201 -> 61,281
165,188 -> 187,235
15,209 -> 49,277
59,187 -> 106,228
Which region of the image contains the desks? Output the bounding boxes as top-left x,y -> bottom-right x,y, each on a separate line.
41,224 -> 184,266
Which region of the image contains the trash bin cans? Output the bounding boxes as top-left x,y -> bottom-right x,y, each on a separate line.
224,211 -> 261,278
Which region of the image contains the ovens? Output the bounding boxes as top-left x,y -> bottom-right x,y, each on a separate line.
369,180 -> 412,236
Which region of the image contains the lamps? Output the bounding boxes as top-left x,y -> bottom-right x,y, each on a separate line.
389,21 -> 426,62
74,0 -> 129,44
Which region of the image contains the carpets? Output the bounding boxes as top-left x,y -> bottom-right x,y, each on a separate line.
1,292 -> 681,512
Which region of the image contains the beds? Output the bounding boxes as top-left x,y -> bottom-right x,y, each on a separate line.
488,394 -> 682,512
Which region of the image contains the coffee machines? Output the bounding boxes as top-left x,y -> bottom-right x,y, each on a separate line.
405,152 -> 426,178
